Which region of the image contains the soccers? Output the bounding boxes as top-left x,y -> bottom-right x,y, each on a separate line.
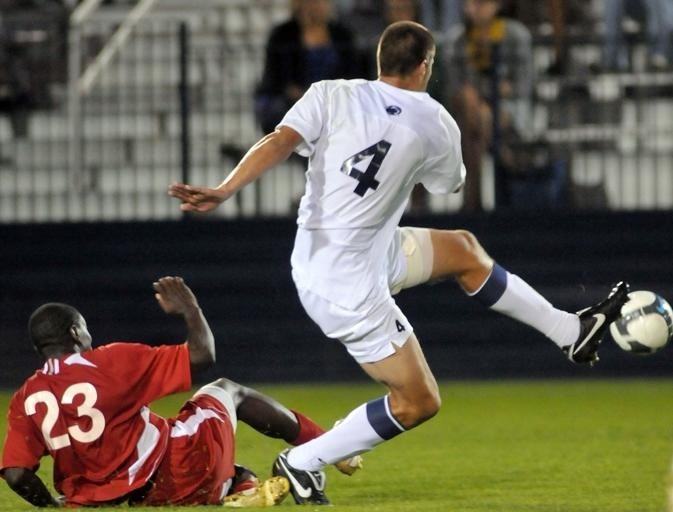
609,290 -> 673,355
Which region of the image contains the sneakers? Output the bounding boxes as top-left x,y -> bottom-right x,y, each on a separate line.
561,279 -> 630,373
223,473 -> 290,508
331,416 -> 363,475
272,445 -> 330,506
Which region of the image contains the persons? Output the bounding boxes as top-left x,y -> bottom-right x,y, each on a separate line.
0,0 -> 79,137
167,19 -> 631,503
0,275 -> 362,508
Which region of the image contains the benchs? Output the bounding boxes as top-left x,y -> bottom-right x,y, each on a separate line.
2,2 -> 673,223
